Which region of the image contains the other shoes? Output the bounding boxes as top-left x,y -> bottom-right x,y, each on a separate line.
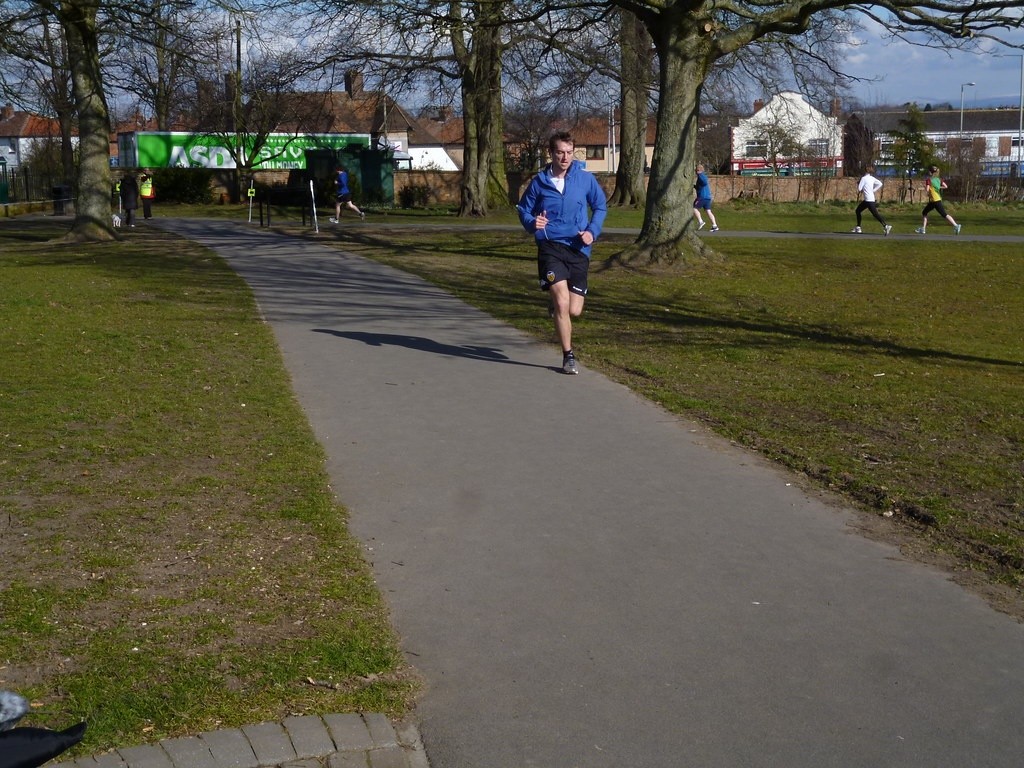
146,217 -> 153,220
131,224 -> 136,228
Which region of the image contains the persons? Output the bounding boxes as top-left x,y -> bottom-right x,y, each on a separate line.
329,167 -> 365,223
520,133 -> 607,376
915,166 -> 961,234
137,168 -> 154,220
121,169 -> 139,228
851,166 -> 892,236
691,164 -> 719,232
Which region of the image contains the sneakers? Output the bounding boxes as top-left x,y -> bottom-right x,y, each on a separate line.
915,227 -> 925,234
954,224 -> 961,235
709,226 -> 719,231
359,212 -> 365,221
851,226 -> 862,233
697,221 -> 705,230
329,218 -> 339,224
548,300 -> 554,317
885,225 -> 892,237
562,355 -> 579,375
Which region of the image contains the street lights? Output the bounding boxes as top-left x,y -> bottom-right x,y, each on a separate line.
993,53 -> 1024,176
961,82 -> 975,137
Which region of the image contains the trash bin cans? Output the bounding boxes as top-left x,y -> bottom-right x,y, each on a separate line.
51,185 -> 71,216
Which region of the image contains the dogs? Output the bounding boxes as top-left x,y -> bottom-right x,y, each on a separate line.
112,214 -> 121,227
